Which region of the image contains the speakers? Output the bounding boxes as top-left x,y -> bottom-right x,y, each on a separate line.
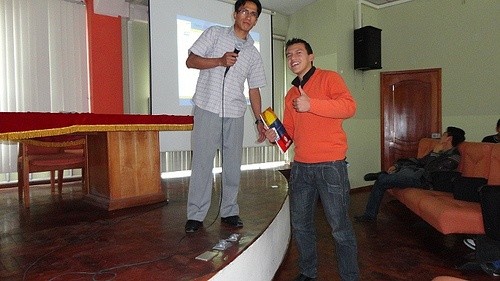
353,25 -> 382,70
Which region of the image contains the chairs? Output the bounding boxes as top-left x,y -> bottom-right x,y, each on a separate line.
18,139 -> 85,208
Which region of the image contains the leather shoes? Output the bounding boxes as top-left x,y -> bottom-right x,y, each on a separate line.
185,220 -> 203,232
221,215 -> 243,227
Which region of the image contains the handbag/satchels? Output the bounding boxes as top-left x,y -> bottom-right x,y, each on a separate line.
431,165 -> 460,192
454,176 -> 488,202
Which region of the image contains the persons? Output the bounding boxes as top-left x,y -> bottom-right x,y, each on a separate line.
354,126 -> 465,225
264,38 -> 360,281
481,119 -> 500,143
185,0 -> 266,235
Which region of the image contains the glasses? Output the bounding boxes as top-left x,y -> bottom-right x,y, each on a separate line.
237,8 -> 258,17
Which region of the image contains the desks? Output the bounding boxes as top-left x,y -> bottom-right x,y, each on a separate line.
0,111 -> 194,210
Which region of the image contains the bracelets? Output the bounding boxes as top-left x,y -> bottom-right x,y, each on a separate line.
255,118 -> 263,124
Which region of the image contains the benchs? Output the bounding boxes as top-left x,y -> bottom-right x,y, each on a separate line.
387,138 -> 500,259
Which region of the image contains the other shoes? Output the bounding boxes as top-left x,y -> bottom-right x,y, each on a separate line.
365,171 -> 387,181
354,215 -> 377,223
464,239 -> 476,251
480,262 -> 500,276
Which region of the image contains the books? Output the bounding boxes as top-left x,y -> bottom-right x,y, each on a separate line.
260,106 -> 293,154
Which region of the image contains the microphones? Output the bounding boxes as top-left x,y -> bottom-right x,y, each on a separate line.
225,43 -> 242,75
